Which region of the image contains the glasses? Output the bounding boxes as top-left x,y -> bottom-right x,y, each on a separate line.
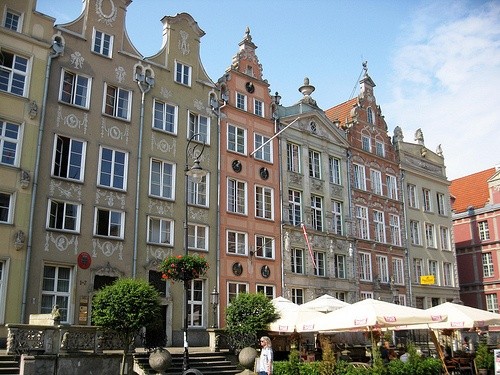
260,341 -> 264,342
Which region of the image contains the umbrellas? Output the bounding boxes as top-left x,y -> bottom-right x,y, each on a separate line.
265,294 -> 500,341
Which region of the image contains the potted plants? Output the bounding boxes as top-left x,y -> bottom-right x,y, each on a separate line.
475,345 -> 494,375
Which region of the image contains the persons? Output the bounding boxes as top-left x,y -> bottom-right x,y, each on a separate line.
258,336 -> 273,375
380,346 -> 410,363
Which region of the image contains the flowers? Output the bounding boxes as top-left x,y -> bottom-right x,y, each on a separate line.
160,252 -> 210,284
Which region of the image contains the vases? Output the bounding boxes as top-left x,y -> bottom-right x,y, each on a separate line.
179,272 -> 194,281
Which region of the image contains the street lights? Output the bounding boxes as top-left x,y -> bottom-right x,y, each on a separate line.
184,133 -> 208,373
